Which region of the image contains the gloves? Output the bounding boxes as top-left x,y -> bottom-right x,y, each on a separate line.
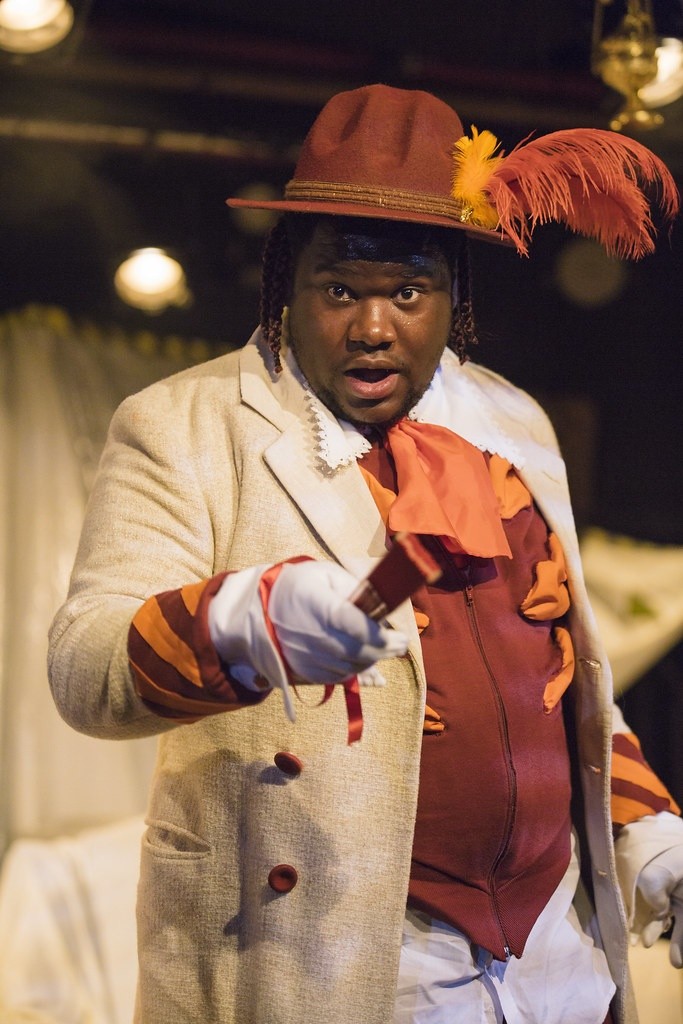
206,556 -> 407,688
636,846 -> 683,969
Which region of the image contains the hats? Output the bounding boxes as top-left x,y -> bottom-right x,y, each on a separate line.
225,83 -> 681,263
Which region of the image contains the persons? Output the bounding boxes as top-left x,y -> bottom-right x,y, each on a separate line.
42,82 -> 683,1024
